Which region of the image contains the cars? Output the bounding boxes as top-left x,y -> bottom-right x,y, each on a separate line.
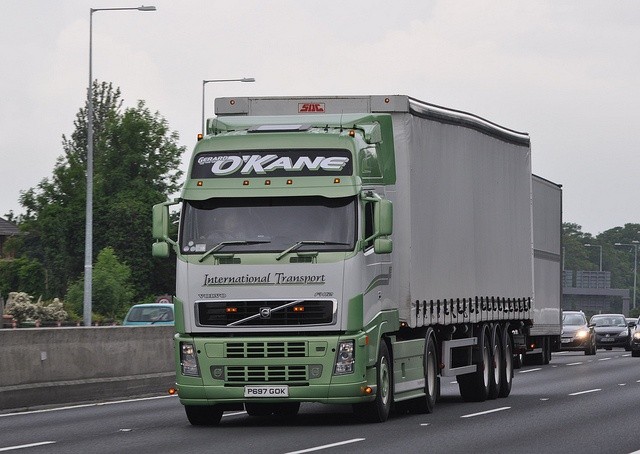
590,314 -> 635,351
631,315 -> 640,357
625,319 -> 637,332
561,310 -> 596,356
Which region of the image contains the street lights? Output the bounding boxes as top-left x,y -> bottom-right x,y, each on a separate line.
84,6 -> 156,327
202,78 -> 255,137
584,244 -> 602,272
615,243 -> 637,308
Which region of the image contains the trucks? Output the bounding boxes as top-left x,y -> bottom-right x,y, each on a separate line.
512,174 -> 563,369
151,95 -> 530,425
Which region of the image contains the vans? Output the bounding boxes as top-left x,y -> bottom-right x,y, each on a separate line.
123,304 -> 175,324
